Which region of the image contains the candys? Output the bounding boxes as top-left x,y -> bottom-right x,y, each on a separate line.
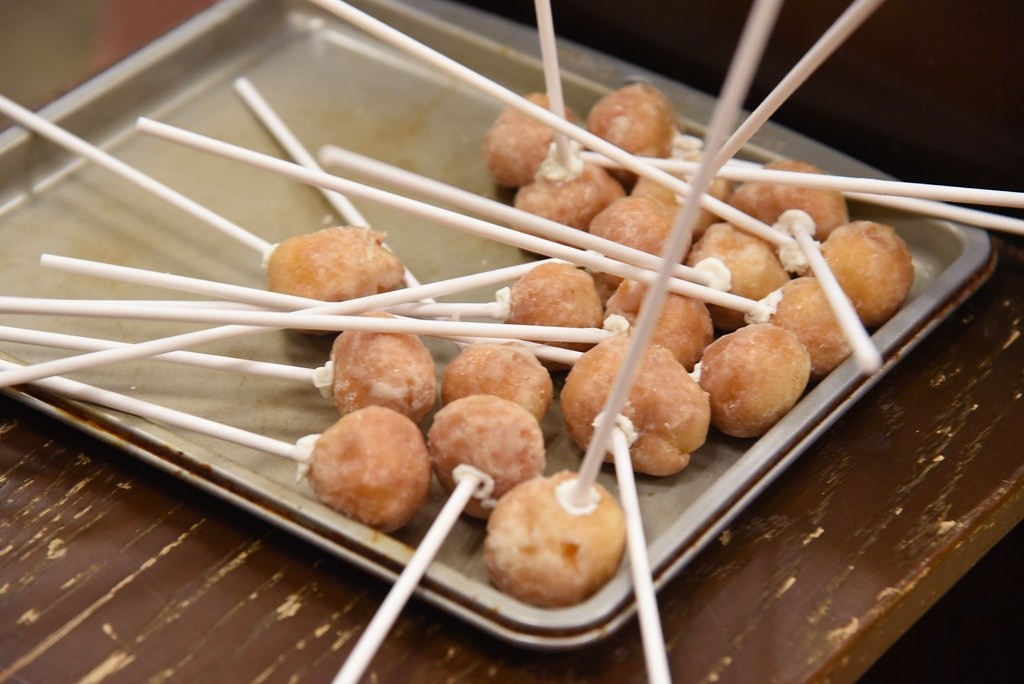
255,85 -> 915,607
